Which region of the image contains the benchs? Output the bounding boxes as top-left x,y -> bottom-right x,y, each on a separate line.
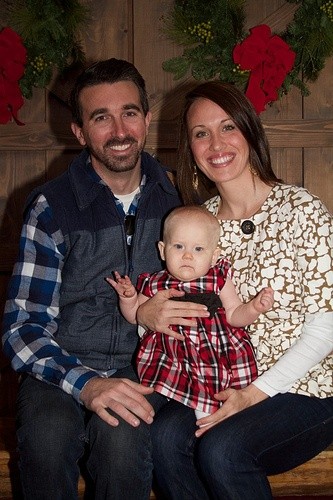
0,443 -> 333,500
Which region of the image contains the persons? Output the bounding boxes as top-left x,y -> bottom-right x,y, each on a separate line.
0,58 -> 183,500
103,206 -> 274,431
147,81 -> 333,500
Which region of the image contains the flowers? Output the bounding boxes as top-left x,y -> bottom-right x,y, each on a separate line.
159,0 -> 333,115
0,0 -> 96,126
